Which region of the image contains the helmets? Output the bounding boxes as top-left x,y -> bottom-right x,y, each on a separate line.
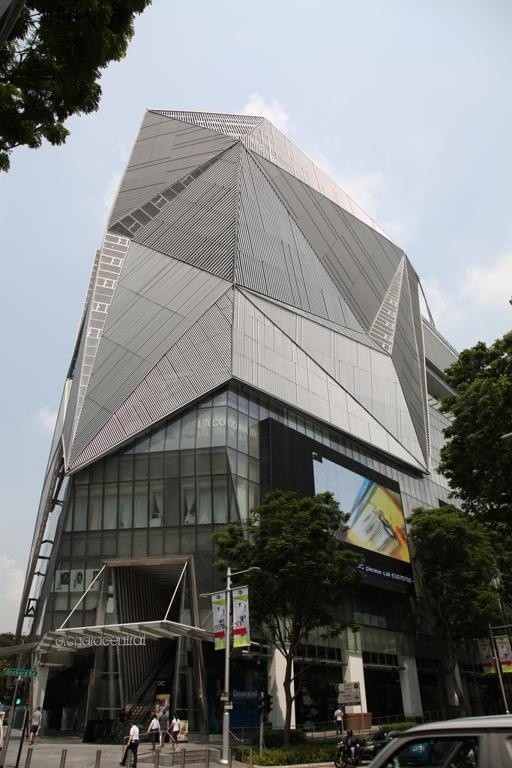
347,729 -> 353,736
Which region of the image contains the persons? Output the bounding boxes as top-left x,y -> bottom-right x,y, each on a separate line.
346,729 -> 356,760
334,706 -> 344,735
148,710 -> 180,750
29,707 -> 43,745
119,720 -> 140,768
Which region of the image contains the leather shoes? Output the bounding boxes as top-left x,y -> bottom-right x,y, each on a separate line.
119,761 -> 137,767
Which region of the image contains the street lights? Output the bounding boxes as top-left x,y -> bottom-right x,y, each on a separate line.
13,636 -> 25,669
199,567 -> 263,765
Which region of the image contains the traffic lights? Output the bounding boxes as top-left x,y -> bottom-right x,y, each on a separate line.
267,695 -> 274,714
15,685 -> 24,706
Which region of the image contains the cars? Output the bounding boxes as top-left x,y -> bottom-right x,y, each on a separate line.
360,714 -> 512,768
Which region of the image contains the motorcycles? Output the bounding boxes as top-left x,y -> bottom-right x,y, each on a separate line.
334,737 -> 366,768
